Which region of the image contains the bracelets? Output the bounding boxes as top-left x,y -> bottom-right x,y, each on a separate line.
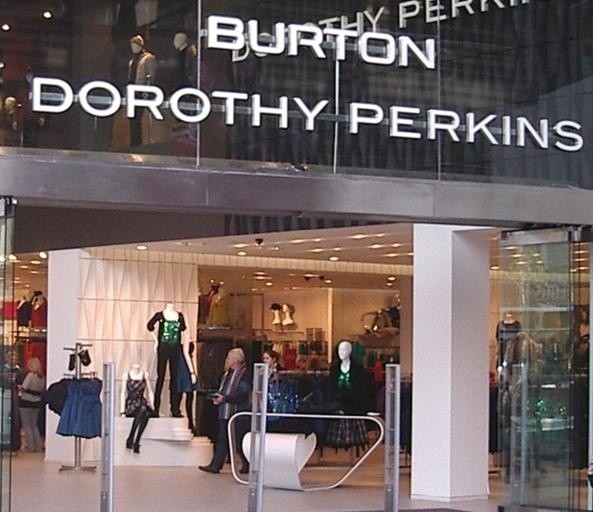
190,372 -> 194,375
120,412 -> 125,415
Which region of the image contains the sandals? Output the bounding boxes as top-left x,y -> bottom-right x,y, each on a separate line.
20,447 -> 40,452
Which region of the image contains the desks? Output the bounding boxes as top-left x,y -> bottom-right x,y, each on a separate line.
242,432 -> 317,491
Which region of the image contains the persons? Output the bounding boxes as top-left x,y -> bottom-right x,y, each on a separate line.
197,347 -> 251,474
29,290 -> 46,329
329,338 -> 371,408
2,351 -> 21,386
223,361 -> 233,465
269,302 -> 281,324
280,302 -> 293,325
494,313 -> 520,364
170,337 -> 197,435
17,357 -> 45,451
146,303 -> 188,417
575,306 -> 589,352
261,349 -> 286,376
119,364 -> 156,453
15,294 -> 30,324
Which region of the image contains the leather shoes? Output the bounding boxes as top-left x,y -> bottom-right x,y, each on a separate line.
198,463 -> 220,473
239,463 -> 249,474
